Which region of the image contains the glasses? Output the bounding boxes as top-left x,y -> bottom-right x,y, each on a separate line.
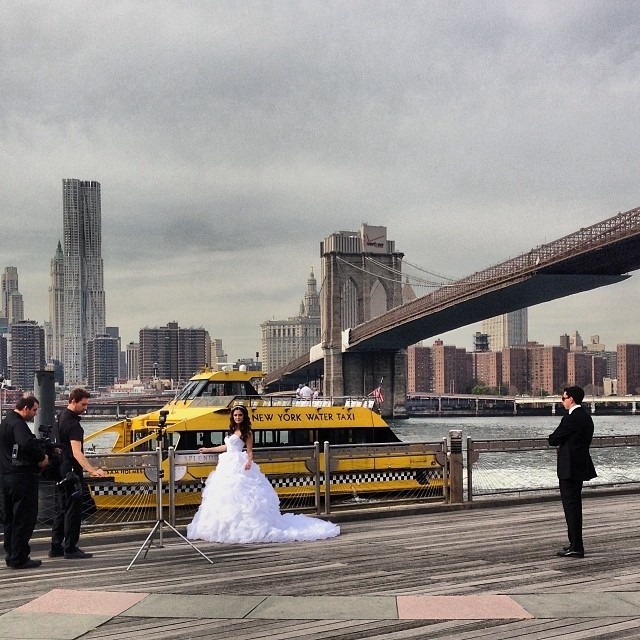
561,395 -> 572,401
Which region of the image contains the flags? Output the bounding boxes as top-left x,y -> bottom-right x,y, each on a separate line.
372,387 -> 384,404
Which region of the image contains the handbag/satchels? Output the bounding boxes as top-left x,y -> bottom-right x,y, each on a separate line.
54,470 -> 83,501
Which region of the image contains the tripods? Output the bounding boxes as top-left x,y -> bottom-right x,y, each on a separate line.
120,447 -> 218,573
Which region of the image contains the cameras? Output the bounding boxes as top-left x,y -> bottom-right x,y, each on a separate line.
154,403 -> 178,430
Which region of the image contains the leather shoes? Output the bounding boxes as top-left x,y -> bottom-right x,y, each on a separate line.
563,546 -> 570,550
10,559 -> 43,569
49,549 -> 64,557
64,548 -> 93,559
557,547 -> 585,557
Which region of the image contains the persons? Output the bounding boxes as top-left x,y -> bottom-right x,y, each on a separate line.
295,381 -> 319,401
1,392 -> 63,570
48,387 -> 107,559
186,405 -> 341,545
547,386 -> 598,559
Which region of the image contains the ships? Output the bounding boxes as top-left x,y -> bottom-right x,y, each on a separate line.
85,370 -> 455,508
